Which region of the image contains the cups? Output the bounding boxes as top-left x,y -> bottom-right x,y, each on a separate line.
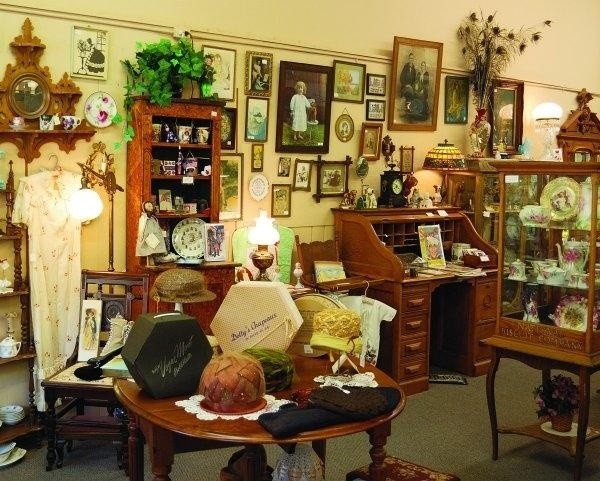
567,263 -> 600,290
39,114 -> 54,131
151,123 -> 211,145
11,116 -> 25,126
60,115 -> 81,131
0,337 -> 22,359
507,258 -> 528,281
528,258 -> 558,273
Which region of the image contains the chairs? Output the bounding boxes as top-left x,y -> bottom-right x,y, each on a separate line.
295,230 -> 386,293
41,269 -> 149,477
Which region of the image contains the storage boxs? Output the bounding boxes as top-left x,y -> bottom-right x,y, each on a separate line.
210,280 -> 304,356
121,311 -> 213,399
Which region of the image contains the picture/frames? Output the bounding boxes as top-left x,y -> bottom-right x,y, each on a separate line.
69,25 -> 109,81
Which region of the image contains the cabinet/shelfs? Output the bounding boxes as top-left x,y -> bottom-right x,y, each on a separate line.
126,95 -> 243,337
478,158 -> 600,481
0,160 -> 46,451
332,207 -> 501,397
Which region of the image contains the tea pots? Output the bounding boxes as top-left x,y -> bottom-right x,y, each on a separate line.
522,297 -> 540,324
535,266 -> 568,288
574,176 -> 600,231
555,237 -> 590,281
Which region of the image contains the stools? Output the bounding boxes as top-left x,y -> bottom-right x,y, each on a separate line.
346,456 -> 462,481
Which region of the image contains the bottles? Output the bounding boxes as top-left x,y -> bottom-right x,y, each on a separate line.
518,205 -> 552,228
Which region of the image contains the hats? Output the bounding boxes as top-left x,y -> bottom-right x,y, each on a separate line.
309,308 -> 363,355
149,269 -> 217,304
198,354 -> 267,415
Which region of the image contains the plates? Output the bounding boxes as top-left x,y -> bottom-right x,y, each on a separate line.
539,177 -> 582,223
0,405 -> 26,428
84,91 -> 118,129
0,440 -> 27,466
8,124 -> 30,131
553,294 -> 600,333
171,217 -> 209,260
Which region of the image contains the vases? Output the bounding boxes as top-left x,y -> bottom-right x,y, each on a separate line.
549,415 -> 575,432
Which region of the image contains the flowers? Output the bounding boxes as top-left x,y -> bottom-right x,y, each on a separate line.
532,374 -> 580,419
457,10 -> 551,111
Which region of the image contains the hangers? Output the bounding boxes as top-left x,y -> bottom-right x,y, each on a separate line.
48,154 -> 63,178
362,280 -> 374,305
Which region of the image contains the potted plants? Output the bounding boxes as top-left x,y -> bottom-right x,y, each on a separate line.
112,35 -> 217,151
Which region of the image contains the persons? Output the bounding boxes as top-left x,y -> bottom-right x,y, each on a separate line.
251,58 -> 266,90
400,52 -> 430,122
289,80 -> 316,141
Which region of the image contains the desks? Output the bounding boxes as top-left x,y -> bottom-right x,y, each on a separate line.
112,355 -> 407,481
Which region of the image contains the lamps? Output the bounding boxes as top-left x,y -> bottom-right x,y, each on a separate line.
532,100 -> 563,159
247,211 -> 280,282
